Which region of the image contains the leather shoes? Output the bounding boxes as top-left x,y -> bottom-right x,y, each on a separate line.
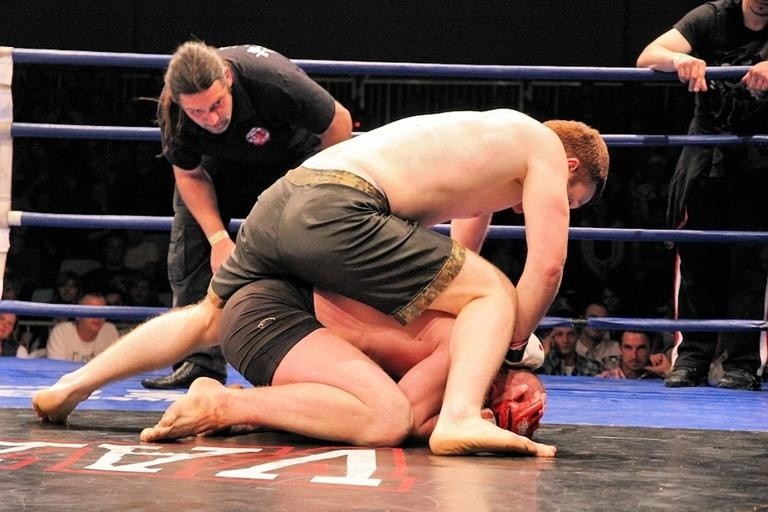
141,362 -> 227,391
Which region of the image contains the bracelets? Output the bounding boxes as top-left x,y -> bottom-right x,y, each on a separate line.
204,230 -> 231,248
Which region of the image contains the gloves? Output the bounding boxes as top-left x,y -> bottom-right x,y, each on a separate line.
489,399 -> 543,438
504,331 -> 546,371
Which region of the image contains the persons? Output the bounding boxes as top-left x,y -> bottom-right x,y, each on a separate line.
139,272 -> 548,449
635,0 -> 768,392
31,106 -> 613,461
137,37 -> 356,392
0,272 -> 766,380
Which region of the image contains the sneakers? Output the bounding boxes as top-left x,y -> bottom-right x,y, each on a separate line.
715,368 -> 762,392
663,364 -> 709,388
